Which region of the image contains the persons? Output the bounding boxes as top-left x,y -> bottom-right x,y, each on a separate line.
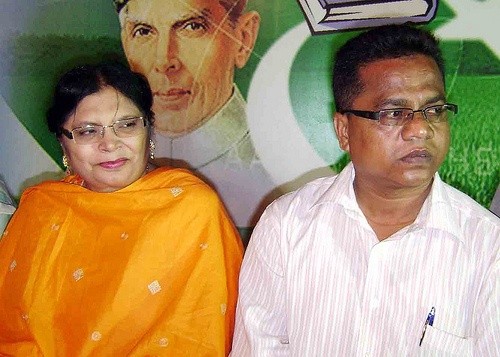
229,24 -> 500,357
114,0 -> 277,227
0,63 -> 246,356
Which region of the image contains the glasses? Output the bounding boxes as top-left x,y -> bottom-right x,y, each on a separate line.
57,113 -> 147,145
339,102 -> 458,126
